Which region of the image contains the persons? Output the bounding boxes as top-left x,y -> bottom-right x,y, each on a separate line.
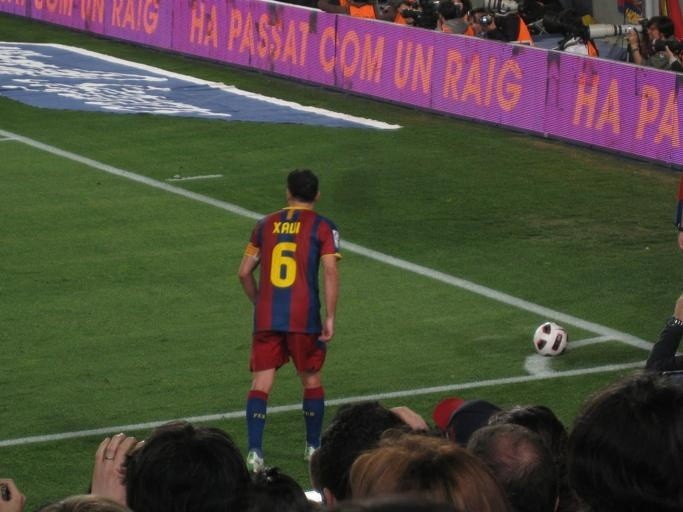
330,0 -> 683,70
89,420 -> 252,510
675,170 -> 683,251
2,479 -> 26,510
41,493 -> 128,511
311,295 -> 683,510
237,166 -> 342,473
248,469 -> 309,510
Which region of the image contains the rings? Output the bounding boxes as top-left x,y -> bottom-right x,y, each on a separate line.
104,457 -> 113,460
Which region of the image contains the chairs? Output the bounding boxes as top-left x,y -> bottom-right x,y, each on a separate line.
244,449 -> 270,476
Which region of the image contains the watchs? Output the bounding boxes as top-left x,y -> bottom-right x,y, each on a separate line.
668,317 -> 682,326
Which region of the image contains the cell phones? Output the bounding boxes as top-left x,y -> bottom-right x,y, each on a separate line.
479,15 -> 492,23
655,39 -> 683,51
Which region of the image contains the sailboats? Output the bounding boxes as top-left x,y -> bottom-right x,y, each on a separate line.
533,321 -> 568,356
